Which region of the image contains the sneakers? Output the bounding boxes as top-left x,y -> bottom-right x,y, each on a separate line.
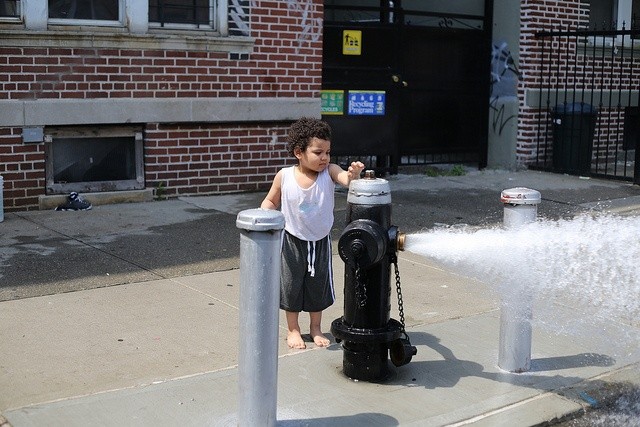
65,191 -> 92,212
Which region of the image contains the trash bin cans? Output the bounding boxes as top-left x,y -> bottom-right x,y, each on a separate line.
552,101 -> 597,175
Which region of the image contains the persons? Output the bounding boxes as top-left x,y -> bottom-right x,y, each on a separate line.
260,116 -> 365,349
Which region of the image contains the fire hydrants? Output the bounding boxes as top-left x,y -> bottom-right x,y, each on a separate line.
328,166 -> 419,384
